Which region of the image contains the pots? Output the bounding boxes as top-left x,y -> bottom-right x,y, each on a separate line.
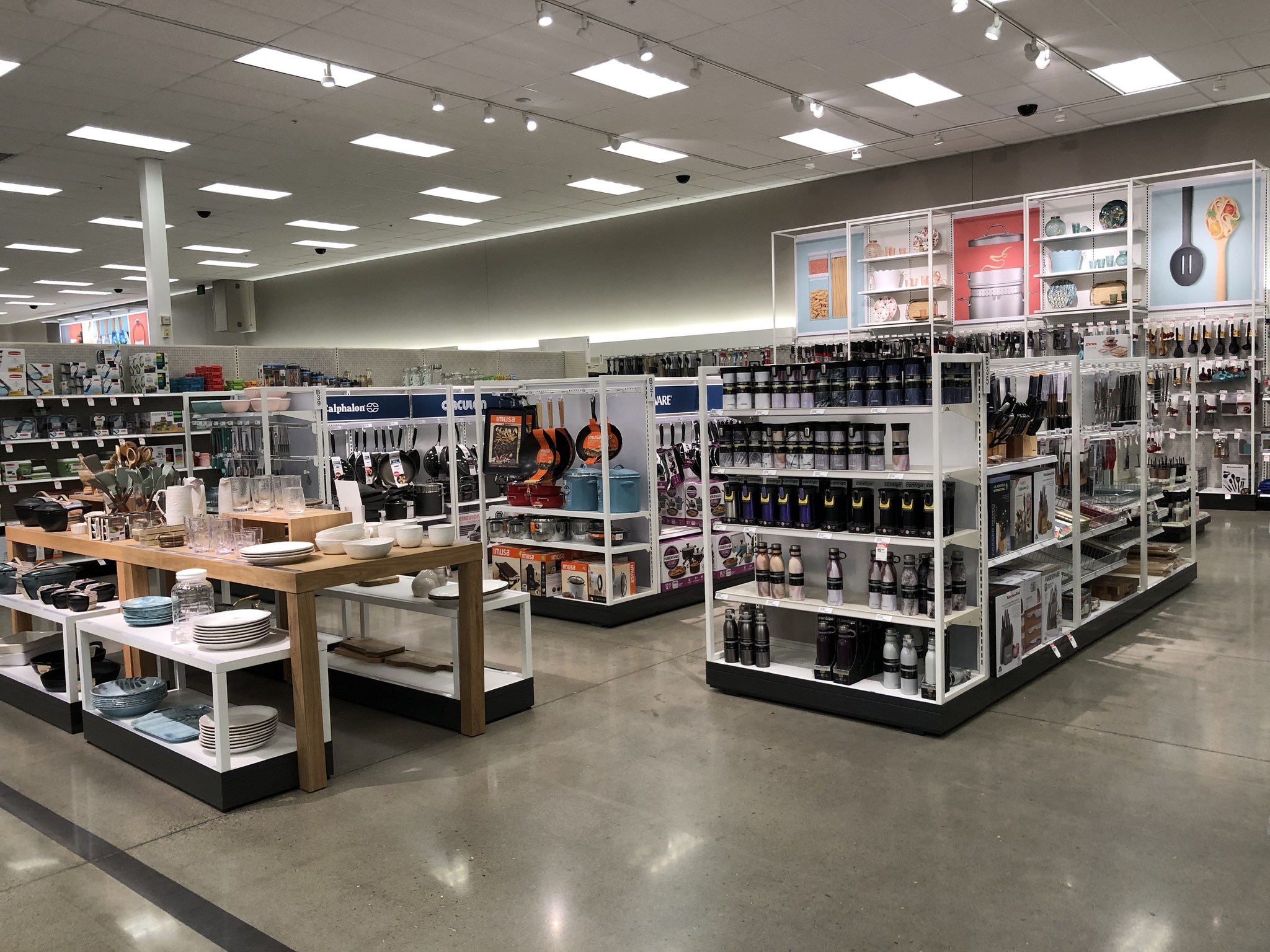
331,395 -> 642,546
0,491 -> 122,691
954,262 -> 1037,319
658,485 -> 756,579
567,571 -> 627,598
656,420 -> 740,489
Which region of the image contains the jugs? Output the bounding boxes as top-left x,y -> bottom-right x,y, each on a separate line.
155,483 -> 196,526
926,271 -> 941,285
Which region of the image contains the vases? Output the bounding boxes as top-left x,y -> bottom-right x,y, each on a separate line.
1045,216 -> 1066,237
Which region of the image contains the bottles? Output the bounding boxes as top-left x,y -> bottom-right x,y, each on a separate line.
864,241 -> 882,258
756,541 -> 967,695
1045,217 -> 1066,236
287,362 -> 516,386
723,604 -> 770,668
170,568 -> 215,645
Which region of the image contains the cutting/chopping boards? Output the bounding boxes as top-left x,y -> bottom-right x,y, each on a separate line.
1062,541 -> 1184,620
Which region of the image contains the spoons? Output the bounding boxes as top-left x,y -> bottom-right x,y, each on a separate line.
1206,195 -> 1240,301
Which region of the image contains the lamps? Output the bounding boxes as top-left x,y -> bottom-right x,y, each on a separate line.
321,63 -> 336,87
983,13 -> 1003,41
934,132 -> 944,146
689,61 -> 704,81
637,38 -> 654,62
577,18 -> 594,43
482,104 -> 496,123
607,135 -> 621,151
1024,37 -> 1051,70
535,2 -> 553,27
951,0 -> 968,13
790,94 -> 826,119
521,115 -> 537,132
805,161 -> 815,169
1213,76 -> 1226,92
430,93 -> 445,111
851,149 -> 862,161
1055,110 -> 1066,123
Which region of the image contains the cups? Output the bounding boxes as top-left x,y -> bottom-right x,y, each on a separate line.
719,362 -> 971,467
230,473 -> 305,517
885,246 -> 928,287
1072,223 -> 1114,269
184,513 -> 263,559
522,584 -> 527,592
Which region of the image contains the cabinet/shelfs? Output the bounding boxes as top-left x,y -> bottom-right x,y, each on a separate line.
770,159 -> 1270,511
192,385 -> 325,514
0,594 -> 119,735
74,612 -> 335,813
474,375 -> 756,629
221,506 -> 353,682
697,356 -> 1196,738
69,491 -> 147,515
0,390 -> 218,582
314,575 -> 534,734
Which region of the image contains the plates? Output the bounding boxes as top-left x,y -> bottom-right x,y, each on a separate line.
90,676 -> 279,754
427,580 -> 509,606
121,596 -> 272,651
912,227 -> 939,252
1099,199 -> 1128,227
238,540 -> 316,566
1047,280 -> 1076,307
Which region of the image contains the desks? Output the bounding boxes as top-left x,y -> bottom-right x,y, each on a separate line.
5,509 -> 485,795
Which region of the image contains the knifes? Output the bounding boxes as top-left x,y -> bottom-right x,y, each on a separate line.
985,369 -> 1137,497
996,483 -> 1058,646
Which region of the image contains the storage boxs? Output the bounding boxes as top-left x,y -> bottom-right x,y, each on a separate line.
124,411 -> 175,434
988,467 -> 1063,680
491,543 -> 636,605
655,476 -> 756,604
1189,467 -> 1207,493
1222,461 -> 1258,495
0,348 -> 170,394
146,443 -> 185,469
0,450 -> 115,482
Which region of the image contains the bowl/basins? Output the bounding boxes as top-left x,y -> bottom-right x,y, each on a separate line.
191,386 -> 291,413
315,519 -> 456,560
562,591 -> 575,599
